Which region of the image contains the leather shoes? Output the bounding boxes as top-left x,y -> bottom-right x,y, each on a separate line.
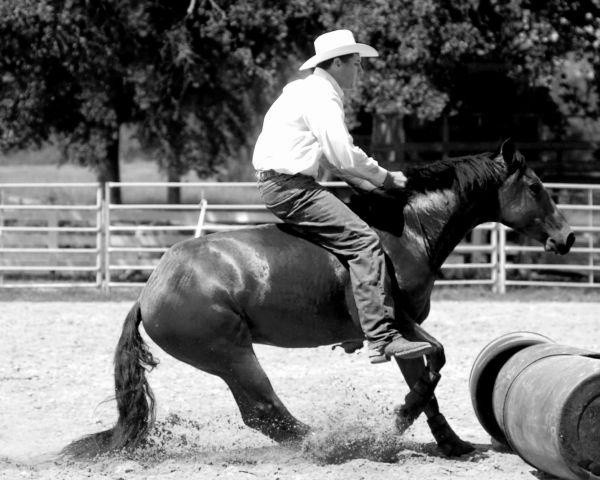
369,334 -> 433,363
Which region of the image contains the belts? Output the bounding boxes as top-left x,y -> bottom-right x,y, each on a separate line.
258,171 -> 283,182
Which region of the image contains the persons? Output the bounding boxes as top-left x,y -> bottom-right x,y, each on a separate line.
252,29 -> 434,364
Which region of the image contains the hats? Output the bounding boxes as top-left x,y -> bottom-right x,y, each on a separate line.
298,28 -> 380,71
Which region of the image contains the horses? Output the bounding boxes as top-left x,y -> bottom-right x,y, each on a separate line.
57,136 -> 575,465
